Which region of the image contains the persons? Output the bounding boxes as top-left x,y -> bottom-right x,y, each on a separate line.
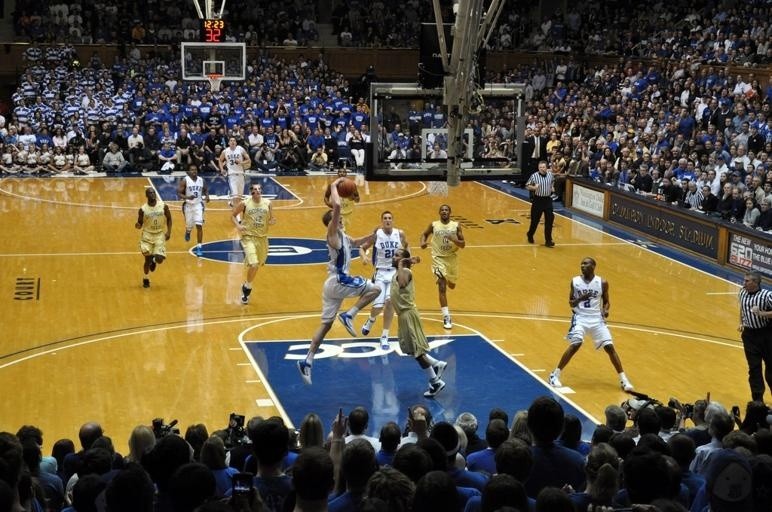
525,161 -> 555,246
549,257 -> 632,393
177,165 -> 210,255
0,395 -> 772,512
0,0 -> 449,178
737,270 -> 772,403
296,176 -> 447,395
468,0 -> 772,234
231,183 -> 277,303
219,137 -> 252,220
134,188 -> 174,287
420,204 -> 465,329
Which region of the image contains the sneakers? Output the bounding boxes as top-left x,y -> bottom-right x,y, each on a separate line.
196,246 -> 202,256
549,373 -> 561,387
149,257 -> 156,272
143,278 -> 149,288
621,378 -> 633,391
338,312 -> 357,337
185,229 -> 191,241
424,361 -> 447,397
362,316 -> 376,335
241,283 -> 252,303
380,335 -> 389,350
444,315 -> 452,328
296,359 -> 312,385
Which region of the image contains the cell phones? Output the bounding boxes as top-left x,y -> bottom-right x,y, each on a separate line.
732,406 -> 740,418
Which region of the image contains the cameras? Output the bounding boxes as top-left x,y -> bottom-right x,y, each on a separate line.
230,414 -> 245,427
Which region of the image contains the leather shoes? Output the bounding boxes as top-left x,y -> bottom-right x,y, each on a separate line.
527,232 -> 534,243
545,242 -> 554,246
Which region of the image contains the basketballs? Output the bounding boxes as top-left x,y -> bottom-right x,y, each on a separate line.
337,178 -> 356,197
